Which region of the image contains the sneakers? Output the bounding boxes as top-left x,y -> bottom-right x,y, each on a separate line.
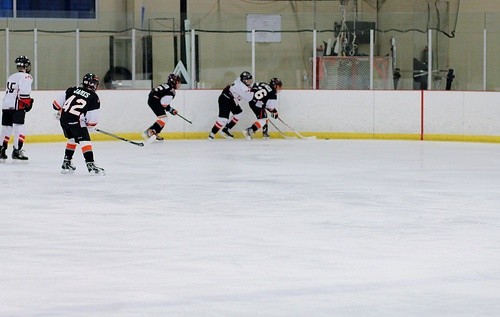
262,133 -> 270,140
11,146 -> 29,164
85,162 -> 105,176
141,129 -> 164,144
208,131 -> 214,142
60,158 -> 77,174
242,128 -> 255,140
0,146 -> 8,164
220,126 -> 234,139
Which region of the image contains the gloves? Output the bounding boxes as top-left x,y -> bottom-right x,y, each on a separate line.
250,82 -> 258,93
166,104 -> 177,116
270,108 -> 279,119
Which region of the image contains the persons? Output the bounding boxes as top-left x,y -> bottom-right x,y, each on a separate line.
208,71 -> 282,140
141,73 -> 180,141
0,55 -> 34,160
59,73 -> 106,176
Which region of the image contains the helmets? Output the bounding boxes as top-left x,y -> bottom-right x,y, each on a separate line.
270,78 -> 282,93
240,71 -> 252,86
83,73 -> 100,91
15,55 -> 31,71
167,74 -> 181,89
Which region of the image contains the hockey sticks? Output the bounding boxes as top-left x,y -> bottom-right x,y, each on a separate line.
277,117 -> 316,140
267,118 -> 297,140
87,126 -> 157,147
175,112 -> 193,124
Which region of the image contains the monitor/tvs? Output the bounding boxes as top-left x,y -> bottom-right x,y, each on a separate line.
333,21 -> 375,43
